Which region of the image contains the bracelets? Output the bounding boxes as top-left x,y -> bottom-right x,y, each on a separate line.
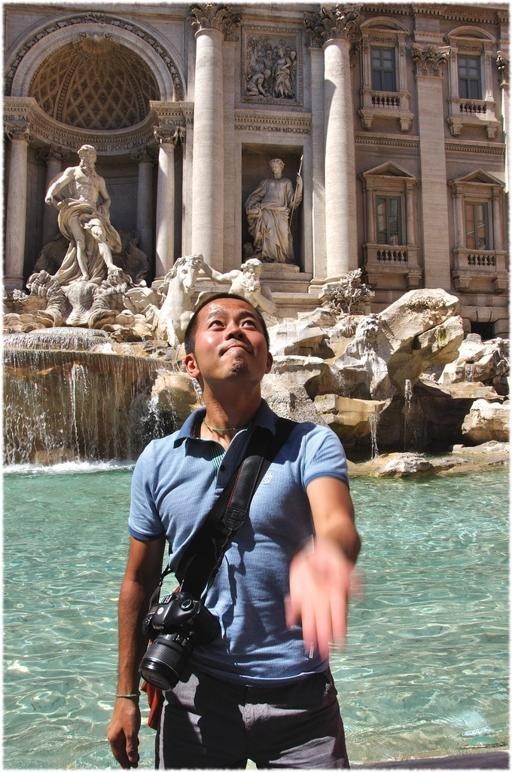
113,693 -> 141,698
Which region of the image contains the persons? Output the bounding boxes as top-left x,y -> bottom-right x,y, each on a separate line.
247,34 -> 298,102
44,142 -> 123,281
193,255 -> 279,315
105,292 -> 364,769
244,158 -> 305,265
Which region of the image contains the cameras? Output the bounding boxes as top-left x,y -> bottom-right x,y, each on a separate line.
136,599 -> 221,691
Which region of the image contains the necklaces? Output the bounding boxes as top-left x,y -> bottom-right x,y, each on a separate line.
199,412 -> 248,433
